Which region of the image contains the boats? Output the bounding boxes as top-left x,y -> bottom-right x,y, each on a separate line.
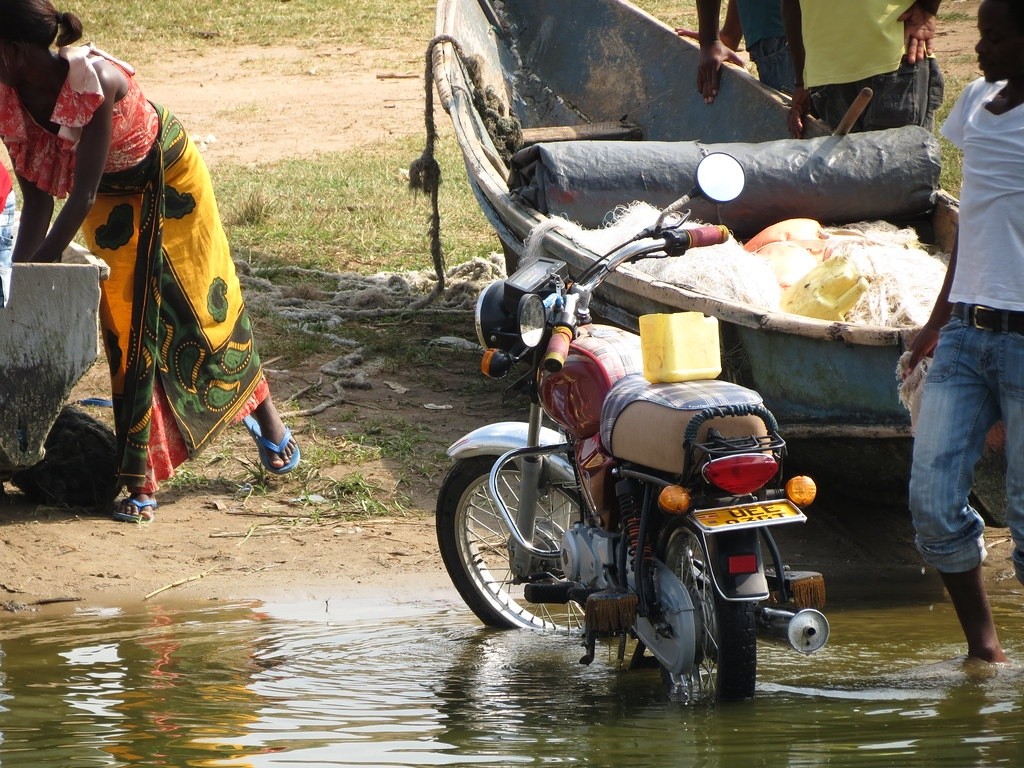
431,0 -> 1008,528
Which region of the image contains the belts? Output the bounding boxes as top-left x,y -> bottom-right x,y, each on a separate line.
951,301 -> 1024,332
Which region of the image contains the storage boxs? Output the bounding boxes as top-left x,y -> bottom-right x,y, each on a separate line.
638,311 -> 723,383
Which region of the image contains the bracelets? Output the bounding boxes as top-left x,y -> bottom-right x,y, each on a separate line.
794,81 -> 804,87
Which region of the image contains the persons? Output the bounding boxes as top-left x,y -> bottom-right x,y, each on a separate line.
674,0 -> 796,105
0,0 -> 300,523
780,0 -> 945,139
901,0 -> 1024,662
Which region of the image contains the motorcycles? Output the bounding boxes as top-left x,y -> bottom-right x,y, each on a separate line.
436,152 -> 831,708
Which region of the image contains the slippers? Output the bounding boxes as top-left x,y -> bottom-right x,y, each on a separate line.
242,414 -> 300,475
112,497 -> 157,524
